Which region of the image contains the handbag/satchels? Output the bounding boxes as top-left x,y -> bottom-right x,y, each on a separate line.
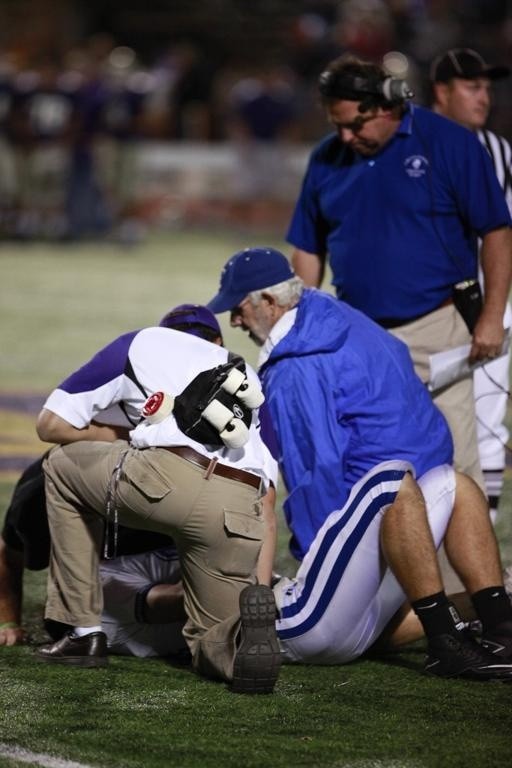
142,355 -> 264,451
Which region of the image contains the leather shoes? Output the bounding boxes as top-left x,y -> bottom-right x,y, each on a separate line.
35,631 -> 109,668
230,585 -> 281,693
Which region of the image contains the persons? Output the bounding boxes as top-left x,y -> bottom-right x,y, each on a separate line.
0,2 -> 511,242
423,49 -> 511,525
283,57 -> 512,514
203,246 -> 512,651
32,302 -> 284,697
0,450 -> 172,655
100,458 -> 511,680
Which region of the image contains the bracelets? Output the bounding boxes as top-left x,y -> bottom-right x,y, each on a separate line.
0,620 -> 18,630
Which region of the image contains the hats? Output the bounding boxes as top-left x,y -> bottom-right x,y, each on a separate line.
158,304 -> 222,338
433,47 -> 510,81
207,247 -> 294,313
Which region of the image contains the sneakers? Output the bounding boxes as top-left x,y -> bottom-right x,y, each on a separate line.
480,629 -> 512,662
422,619 -> 512,681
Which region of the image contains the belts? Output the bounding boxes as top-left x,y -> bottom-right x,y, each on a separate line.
157,446 -> 260,492
